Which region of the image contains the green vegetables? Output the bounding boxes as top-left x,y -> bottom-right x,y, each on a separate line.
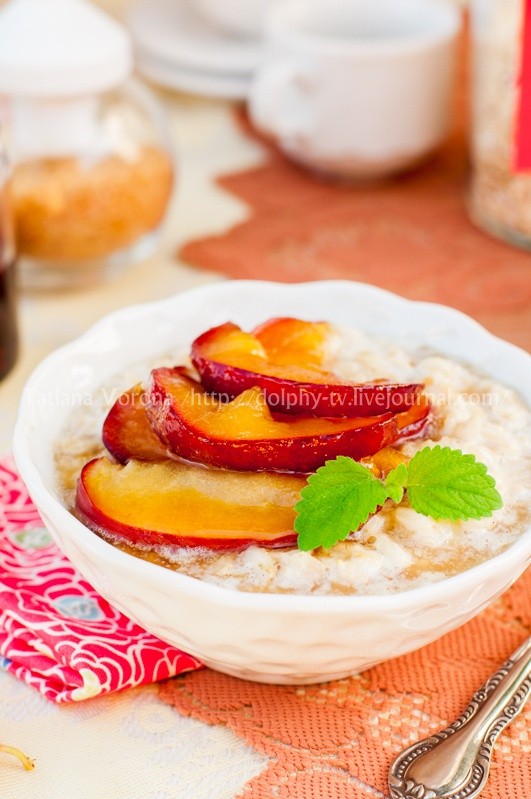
294,445 -> 503,551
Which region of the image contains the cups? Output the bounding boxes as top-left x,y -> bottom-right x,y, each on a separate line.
468,0 -> 531,248
246,2 -> 463,184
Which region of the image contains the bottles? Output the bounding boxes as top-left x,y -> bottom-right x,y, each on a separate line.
0,2 -> 178,287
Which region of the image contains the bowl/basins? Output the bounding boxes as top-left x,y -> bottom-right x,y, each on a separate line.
15,279 -> 530,686
